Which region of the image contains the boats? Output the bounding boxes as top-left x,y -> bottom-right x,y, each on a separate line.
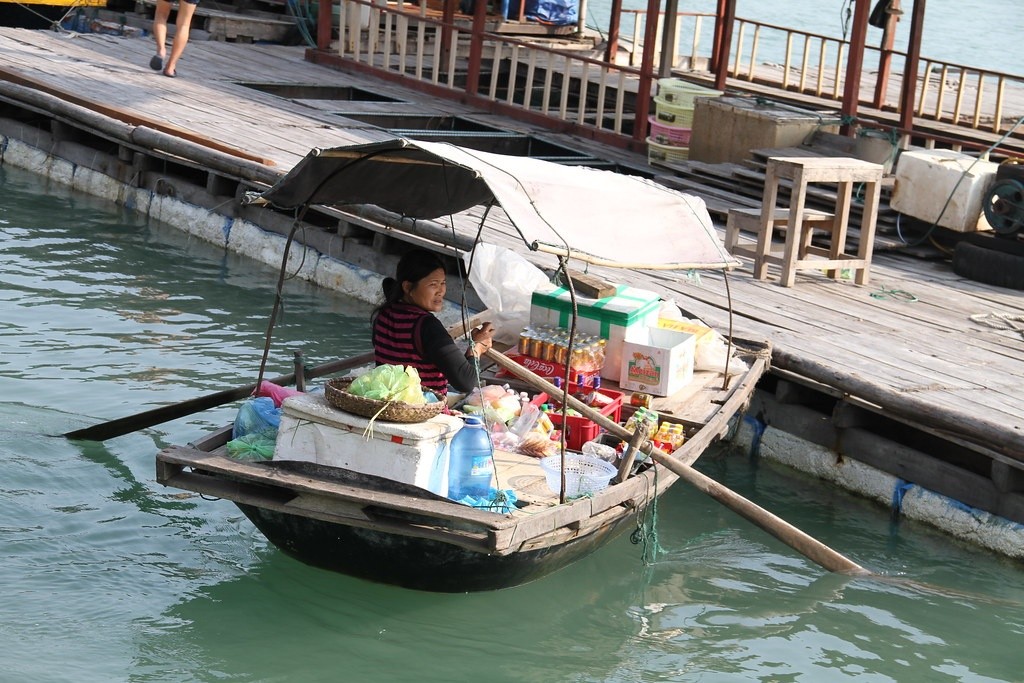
62,137 -> 874,591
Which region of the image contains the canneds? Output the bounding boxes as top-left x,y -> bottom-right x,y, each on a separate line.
630,392 -> 654,409
587,391 -> 614,408
518,324 -> 606,372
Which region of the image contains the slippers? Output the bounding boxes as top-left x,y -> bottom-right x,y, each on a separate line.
149,55 -> 163,71
163,66 -> 177,78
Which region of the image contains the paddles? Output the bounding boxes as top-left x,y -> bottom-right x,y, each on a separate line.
62,317 -> 482,441
482,345 -> 868,575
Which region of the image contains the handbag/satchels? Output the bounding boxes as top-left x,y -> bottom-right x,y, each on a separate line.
227,394 -> 282,460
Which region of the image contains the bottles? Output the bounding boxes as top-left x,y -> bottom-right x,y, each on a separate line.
448,413 -> 493,503
506,373 -> 602,439
624,406 -> 684,450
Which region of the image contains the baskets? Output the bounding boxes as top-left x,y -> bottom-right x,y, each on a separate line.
539,454 -> 618,499
653,96 -> 695,128
645,136 -> 690,166
324,376 -> 447,423
657,77 -> 725,108
647,115 -> 693,145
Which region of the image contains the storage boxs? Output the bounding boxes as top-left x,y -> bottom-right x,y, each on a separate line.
499,282 -> 697,451
690,97 -> 841,164
889,147 -> 1001,231
274,396 -> 464,495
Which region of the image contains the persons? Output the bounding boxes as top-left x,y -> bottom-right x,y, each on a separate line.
149,0 -> 199,77
369,250 -> 496,399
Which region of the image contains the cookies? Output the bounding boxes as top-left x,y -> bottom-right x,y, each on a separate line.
519,438 -> 548,458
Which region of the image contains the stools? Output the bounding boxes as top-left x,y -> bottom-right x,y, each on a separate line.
753,153 -> 886,287
721,208 -> 836,273
